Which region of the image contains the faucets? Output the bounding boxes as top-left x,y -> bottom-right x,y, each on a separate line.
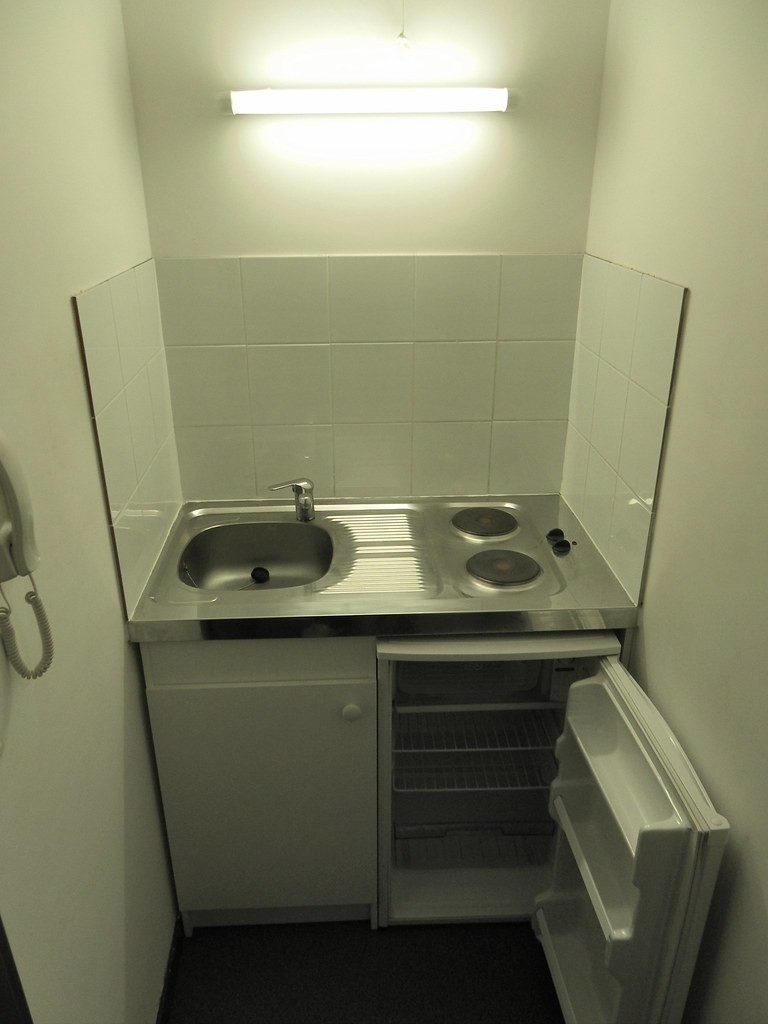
268,478 -> 316,523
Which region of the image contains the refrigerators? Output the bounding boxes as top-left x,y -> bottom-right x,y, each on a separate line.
375,632 -> 731,1023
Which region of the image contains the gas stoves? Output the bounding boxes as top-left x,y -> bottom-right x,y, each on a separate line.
426,493 -> 637,636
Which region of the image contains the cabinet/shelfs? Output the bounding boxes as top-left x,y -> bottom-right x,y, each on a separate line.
136,635 -> 379,934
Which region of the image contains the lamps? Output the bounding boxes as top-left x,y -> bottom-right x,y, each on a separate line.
216,83 -> 513,123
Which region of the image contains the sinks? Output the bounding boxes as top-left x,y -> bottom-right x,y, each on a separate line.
177,521 -> 335,592
